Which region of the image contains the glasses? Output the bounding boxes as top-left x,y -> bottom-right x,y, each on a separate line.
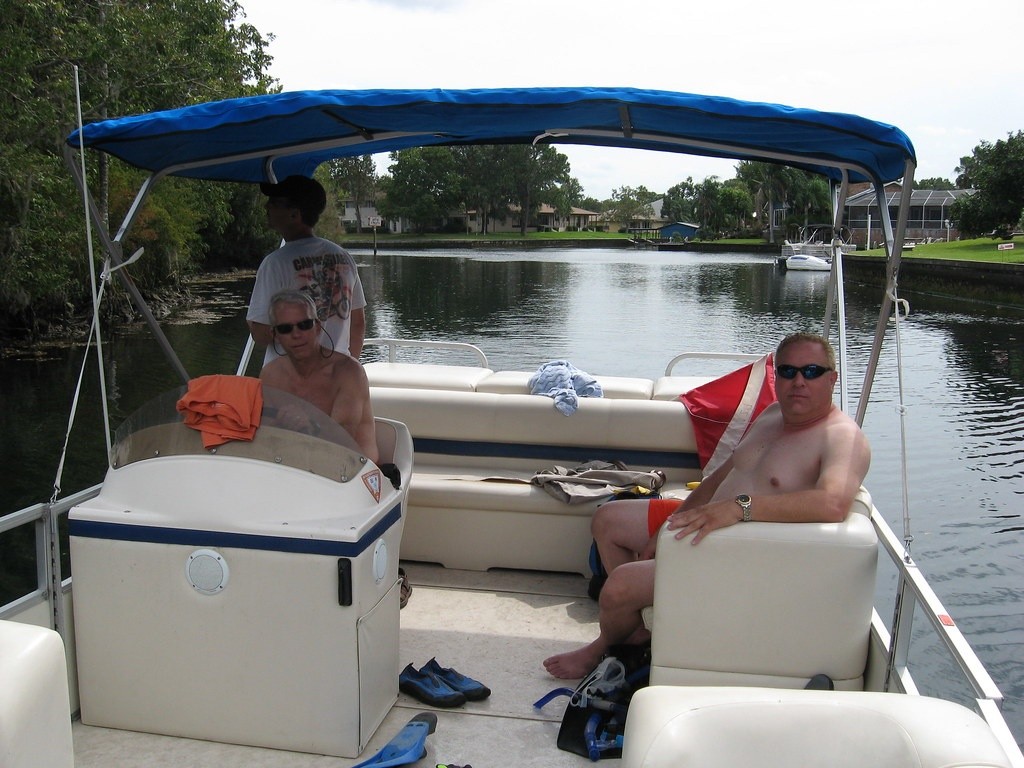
265,194 -> 296,209
272,319 -> 317,334
776,362 -> 831,381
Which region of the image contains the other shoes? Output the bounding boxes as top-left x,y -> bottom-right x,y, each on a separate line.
398,661 -> 466,708
599,640 -> 651,671
420,656 -> 491,702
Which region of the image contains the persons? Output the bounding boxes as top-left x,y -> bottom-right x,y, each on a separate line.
543,332 -> 873,680
248,176 -> 366,365
259,290 -> 377,467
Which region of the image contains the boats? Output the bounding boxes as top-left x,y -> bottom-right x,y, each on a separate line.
777,254 -> 832,271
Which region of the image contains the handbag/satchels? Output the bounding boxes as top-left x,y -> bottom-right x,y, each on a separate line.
557,658 -> 653,760
532,456 -> 668,505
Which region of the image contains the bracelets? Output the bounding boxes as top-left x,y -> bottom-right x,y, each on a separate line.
310,418 -> 321,436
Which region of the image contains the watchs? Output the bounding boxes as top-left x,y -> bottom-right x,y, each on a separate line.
735,493 -> 753,522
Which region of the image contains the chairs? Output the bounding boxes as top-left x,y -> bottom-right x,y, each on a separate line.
372,416 -> 416,537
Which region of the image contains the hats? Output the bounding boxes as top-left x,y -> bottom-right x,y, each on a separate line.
258,174 -> 327,214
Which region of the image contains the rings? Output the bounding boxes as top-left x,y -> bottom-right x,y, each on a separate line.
296,415 -> 300,420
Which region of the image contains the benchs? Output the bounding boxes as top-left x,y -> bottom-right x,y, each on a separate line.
371,387 -> 1010,768
